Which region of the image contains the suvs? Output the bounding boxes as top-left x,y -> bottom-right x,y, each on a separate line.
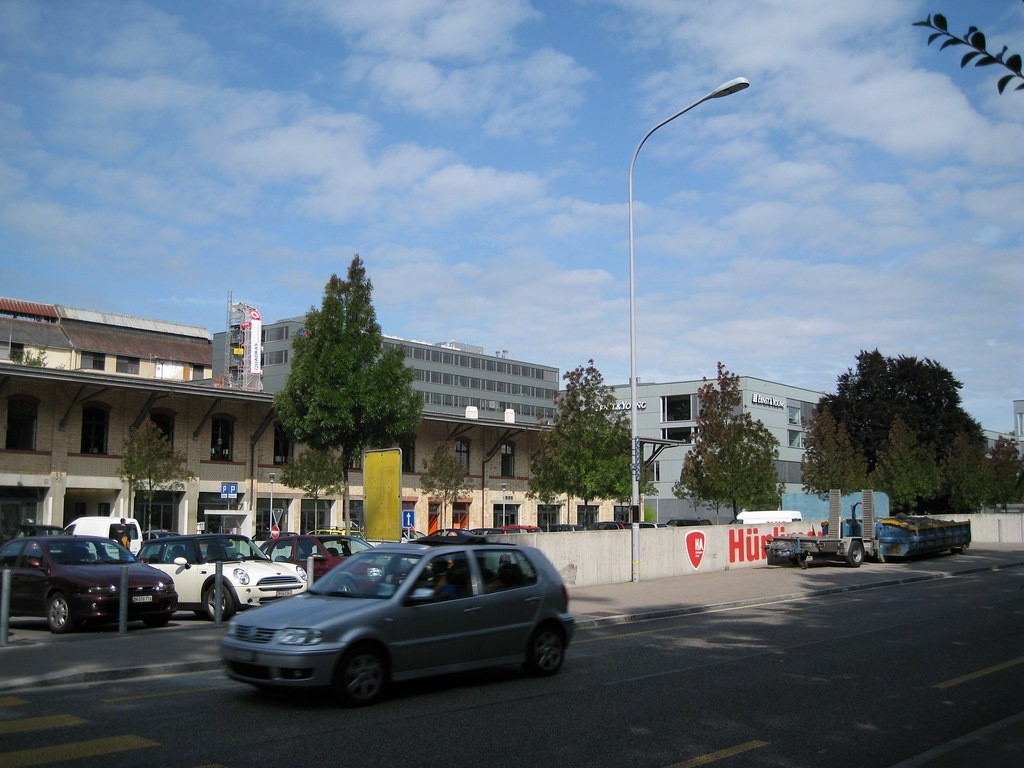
306,526 -> 366,553
133,532 -> 307,623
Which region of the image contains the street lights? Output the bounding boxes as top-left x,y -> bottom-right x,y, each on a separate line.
268,473 -> 276,538
630,74 -> 751,582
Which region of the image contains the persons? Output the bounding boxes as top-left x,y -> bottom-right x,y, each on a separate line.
414,558 -> 455,603
113,518 -> 131,560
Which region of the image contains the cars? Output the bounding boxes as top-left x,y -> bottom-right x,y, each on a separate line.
0,535 -> 180,633
1,525 -> 72,554
409,518 -> 714,557
254,535 -> 384,597
218,526 -> 577,710
141,529 -> 187,552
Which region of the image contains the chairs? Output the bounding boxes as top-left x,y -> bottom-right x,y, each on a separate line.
169,546 -> 190,561
300,543 -> 313,559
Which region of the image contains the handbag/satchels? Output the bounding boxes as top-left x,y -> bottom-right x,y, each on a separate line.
121,536 -> 130,548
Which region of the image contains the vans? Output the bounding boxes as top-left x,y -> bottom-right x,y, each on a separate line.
61,516 -> 143,559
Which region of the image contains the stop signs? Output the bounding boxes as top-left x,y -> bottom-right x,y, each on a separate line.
271,526 -> 280,539
